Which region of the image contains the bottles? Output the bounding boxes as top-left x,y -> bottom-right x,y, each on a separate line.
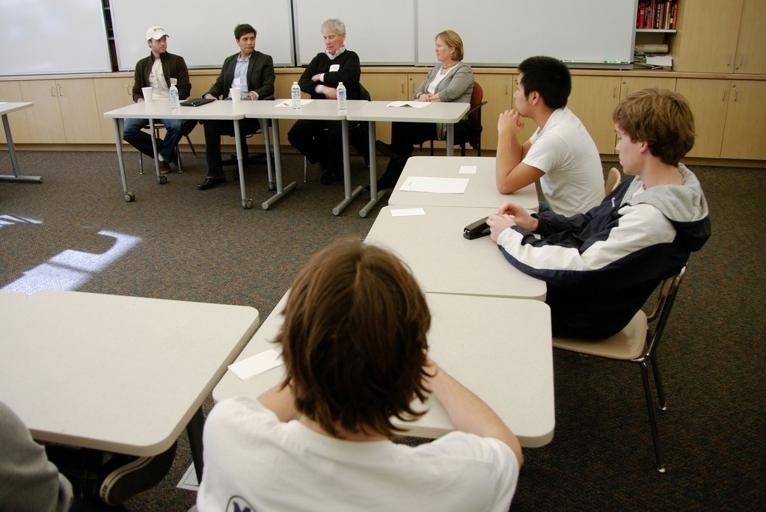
290,81 -> 301,111
169,83 -> 180,110
336,81 -> 347,110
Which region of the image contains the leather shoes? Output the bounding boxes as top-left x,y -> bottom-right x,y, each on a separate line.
197,176 -> 226,189
321,160 -> 343,184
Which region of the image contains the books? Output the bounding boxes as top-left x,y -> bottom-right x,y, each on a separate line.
633,0 -> 680,71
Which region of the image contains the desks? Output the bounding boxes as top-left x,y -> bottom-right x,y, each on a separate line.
212,286 -> 557,450
102,96 -> 276,210
0,100 -> 44,187
346,99 -> 471,218
362,203 -> 549,302
0,291 -> 262,486
245,99 -> 372,217
388,153 -> 540,214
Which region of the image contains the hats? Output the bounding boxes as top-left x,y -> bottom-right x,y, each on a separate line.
147,26 -> 169,41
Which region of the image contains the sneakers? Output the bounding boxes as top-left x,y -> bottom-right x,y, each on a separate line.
157,152 -> 183,174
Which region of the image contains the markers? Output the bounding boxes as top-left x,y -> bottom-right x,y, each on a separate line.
604,60 -> 634,65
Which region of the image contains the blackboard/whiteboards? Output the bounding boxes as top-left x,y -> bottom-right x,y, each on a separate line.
0,0 -> 113,76
109,0 -> 295,72
292,0 -> 415,67
415,0 -> 639,70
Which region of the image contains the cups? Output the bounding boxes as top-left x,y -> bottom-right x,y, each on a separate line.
141,87 -> 153,103
229,87 -> 241,104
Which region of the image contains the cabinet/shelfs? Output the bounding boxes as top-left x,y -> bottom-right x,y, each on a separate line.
20,75 -> 108,146
0,73 -> 25,147
358,66 -> 472,151
93,76 -> 139,146
471,68 -> 539,150
219,73 -> 312,146
157,71 -> 219,147
565,0 -> 765,161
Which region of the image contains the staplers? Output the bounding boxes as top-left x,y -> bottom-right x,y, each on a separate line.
463,216 -> 491,240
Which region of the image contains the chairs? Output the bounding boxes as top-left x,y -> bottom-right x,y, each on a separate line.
419,82 -> 485,157
138,122 -> 197,175
552,261 -> 690,478
606,167 -> 622,194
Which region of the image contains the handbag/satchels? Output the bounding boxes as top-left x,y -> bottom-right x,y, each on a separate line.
179,98 -> 216,107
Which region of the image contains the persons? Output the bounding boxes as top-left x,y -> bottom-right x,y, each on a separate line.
365,28 -> 475,192
286,19 -> 370,186
485,87 -> 710,345
189,236 -> 525,512
0,401 -> 179,510
196,24 -> 276,192
123,26 -> 193,174
495,55 -> 606,217
